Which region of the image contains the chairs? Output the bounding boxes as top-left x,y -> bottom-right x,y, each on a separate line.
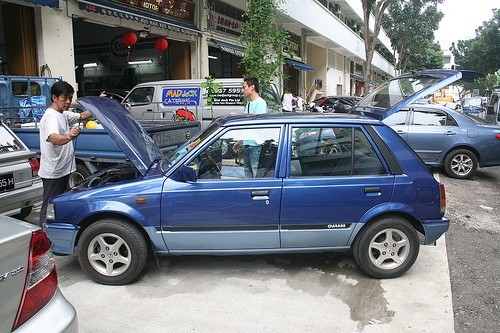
329,149 -> 368,176
351,155 -> 385,176
255,149 -> 277,179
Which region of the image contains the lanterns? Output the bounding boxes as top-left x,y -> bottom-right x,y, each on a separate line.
155,37 -> 168,53
122,28 -> 137,48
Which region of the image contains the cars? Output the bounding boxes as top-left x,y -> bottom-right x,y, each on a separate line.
42,68 -> 484,285
77,88 -> 130,104
0,120 -> 79,333
303,89 -> 500,179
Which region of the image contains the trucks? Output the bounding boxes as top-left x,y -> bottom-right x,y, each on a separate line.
0,72 -> 202,193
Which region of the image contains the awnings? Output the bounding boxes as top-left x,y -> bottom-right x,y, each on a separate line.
351,74 -> 365,82
284,57 -> 314,71
24,0 -> 59,9
75,0 -> 202,38
210,38 -> 248,57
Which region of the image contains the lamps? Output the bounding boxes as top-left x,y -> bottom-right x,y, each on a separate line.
128,59 -> 153,65
83,62 -> 99,67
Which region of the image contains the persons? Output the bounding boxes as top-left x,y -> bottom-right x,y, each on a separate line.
39,81 -> 107,230
233,76 -> 267,178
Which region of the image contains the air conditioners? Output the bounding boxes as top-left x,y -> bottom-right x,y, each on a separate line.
377,43 -> 382,52
350,19 -> 357,29
334,3 -> 342,15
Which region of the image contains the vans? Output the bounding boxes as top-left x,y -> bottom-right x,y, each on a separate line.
120,78 -> 283,159
